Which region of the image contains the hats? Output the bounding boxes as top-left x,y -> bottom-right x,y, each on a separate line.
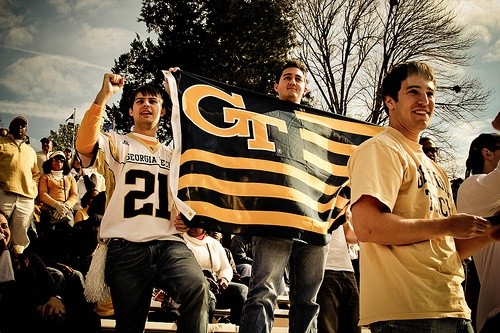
49,150 -> 66,162
40,136 -> 54,142
63,146 -> 72,153
9,115 -> 29,126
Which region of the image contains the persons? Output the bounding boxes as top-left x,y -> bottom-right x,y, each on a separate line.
0,57 -> 500,333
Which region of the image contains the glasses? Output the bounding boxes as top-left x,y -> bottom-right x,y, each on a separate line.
52,157 -> 65,162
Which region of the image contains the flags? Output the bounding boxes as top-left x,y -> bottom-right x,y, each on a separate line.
65,113 -> 74,122
162,69 -> 385,247
